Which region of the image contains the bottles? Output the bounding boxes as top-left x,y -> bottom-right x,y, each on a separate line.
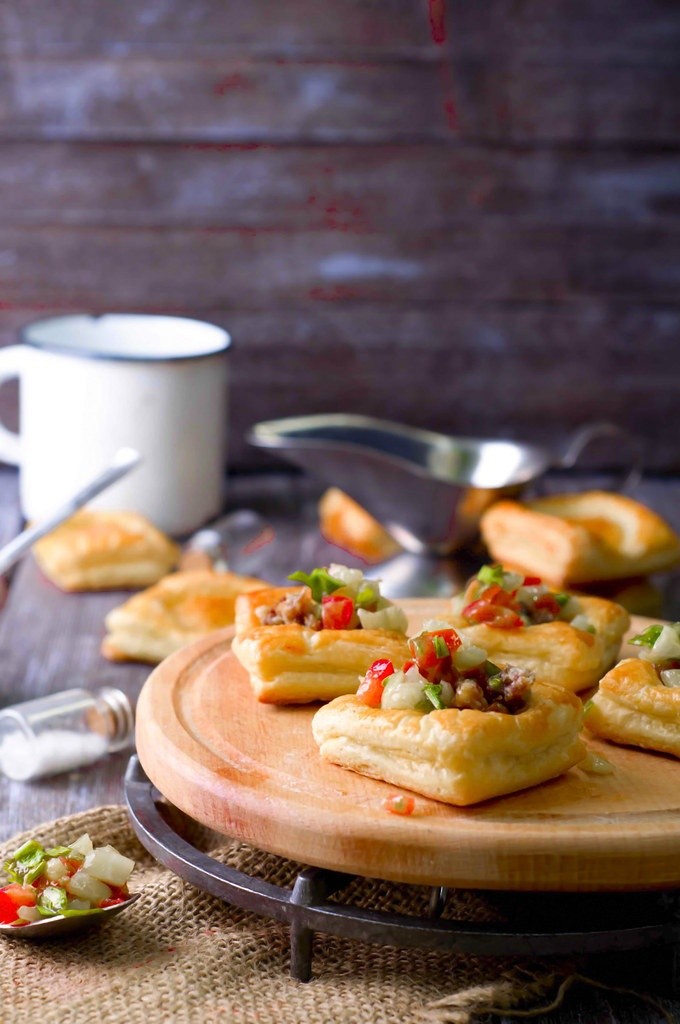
1,687 -> 133,782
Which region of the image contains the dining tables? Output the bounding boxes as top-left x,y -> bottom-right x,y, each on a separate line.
0,467 -> 680,1024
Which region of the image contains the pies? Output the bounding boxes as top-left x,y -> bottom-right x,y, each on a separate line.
32,487 -> 680,806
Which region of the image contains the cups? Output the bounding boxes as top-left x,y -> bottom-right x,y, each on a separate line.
0,315 -> 234,536
244,414 -> 624,600
